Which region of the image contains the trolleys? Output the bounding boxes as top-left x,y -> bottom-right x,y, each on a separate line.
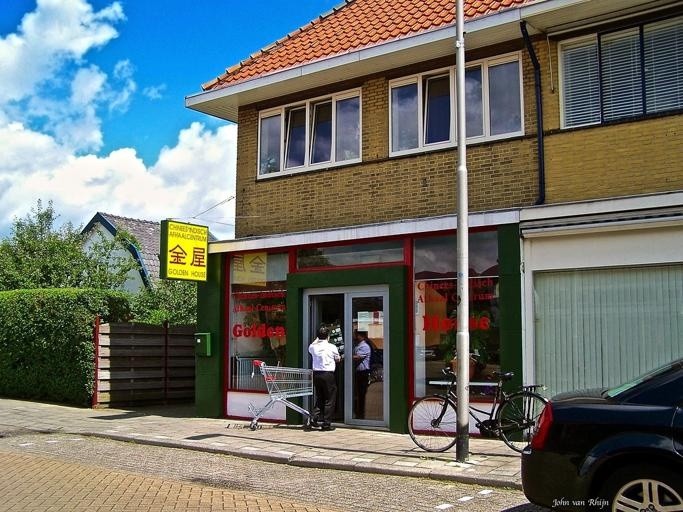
249,360 -> 315,431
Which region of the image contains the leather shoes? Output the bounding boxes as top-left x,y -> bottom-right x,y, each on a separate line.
306,417 -> 319,427
321,426 -> 336,431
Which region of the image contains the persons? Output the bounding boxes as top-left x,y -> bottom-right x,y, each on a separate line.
352,328 -> 371,419
304,325 -> 341,431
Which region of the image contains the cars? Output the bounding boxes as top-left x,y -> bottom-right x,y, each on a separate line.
370,363 -> 383,383
417,348 -> 437,361
521,357 -> 683,512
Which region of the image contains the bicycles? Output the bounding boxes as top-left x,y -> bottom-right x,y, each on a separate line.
408,367 -> 548,453
